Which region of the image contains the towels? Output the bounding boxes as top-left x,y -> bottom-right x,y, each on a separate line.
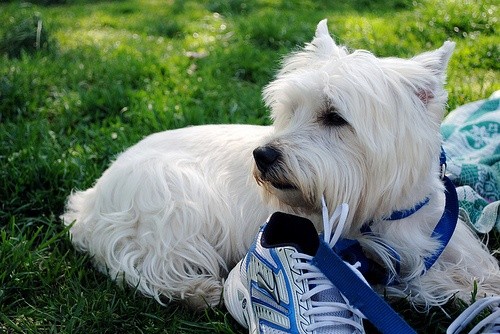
439,88 -> 500,234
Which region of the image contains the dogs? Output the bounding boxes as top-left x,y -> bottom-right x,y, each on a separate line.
59,18 -> 500,316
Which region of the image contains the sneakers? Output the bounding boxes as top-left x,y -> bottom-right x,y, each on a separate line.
223,212 -> 364,334
